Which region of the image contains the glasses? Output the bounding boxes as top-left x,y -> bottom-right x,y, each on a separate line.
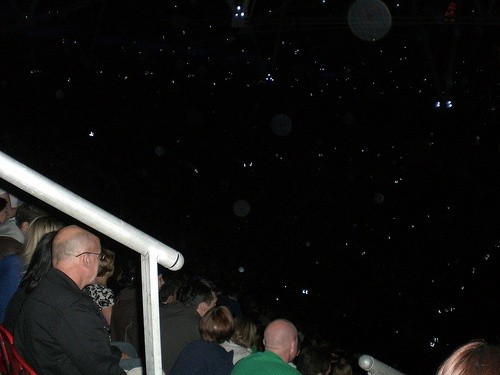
76,252 -> 105,260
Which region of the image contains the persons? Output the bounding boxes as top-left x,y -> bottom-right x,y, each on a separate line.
434,342 -> 500,375
0,182 -> 357,375
